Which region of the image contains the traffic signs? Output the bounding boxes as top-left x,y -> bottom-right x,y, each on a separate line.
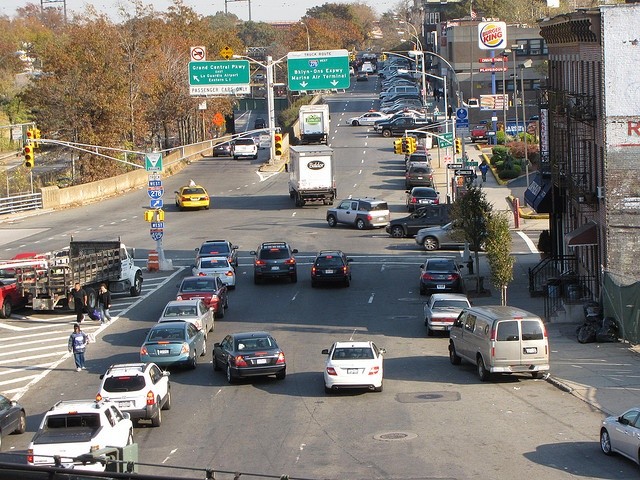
288,49 -> 350,91
188,60 -> 250,95
437,132 -> 453,149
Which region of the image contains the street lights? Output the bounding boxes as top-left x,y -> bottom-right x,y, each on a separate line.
232,52 -> 287,162
501,48 -> 512,133
511,43 -> 524,135
0,50 -> 26,66
393,14 -> 461,137
518,58 -> 533,188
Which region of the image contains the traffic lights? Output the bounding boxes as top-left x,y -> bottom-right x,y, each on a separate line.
456,140 -> 460,154
405,139 -> 411,154
26,130 -> 35,148
24,145 -> 34,170
275,133 -> 282,156
35,127 -> 41,148
393,139 -> 401,154
413,137 -> 417,152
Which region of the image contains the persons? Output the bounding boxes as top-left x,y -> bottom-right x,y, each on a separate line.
69,283 -> 87,324
433,88 -> 437,101
469,159 -> 475,174
433,105 -> 439,121
68,324 -> 90,372
99,284 -> 112,325
436,90 -> 440,102
479,161 -> 491,182
447,105 -> 453,120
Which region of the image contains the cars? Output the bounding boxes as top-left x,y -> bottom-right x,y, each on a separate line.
416,216 -> 488,252
405,165 -> 433,190
421,293 -> 472,336
344,51 -> 433,137
157,299 -> 215,342
599,407 -> 640,466
212,331 -> 287,384
194,239 -> 239,267
416,145 -> 427,150
419,258 -> 463,295
0,394 -> 26,448
404,186 -> 440,212
385,204 -> 453,238
322,341 -> 386,393
213,141 -> 235,157
174,180 -> 210,211
233,138 -> 258,159
470,124 -> 489,143
175,275 -> 229,318
413,150 -> 429,154
139,321 -> 207,370
191,256 -> 236,290
311,250 -> 353,288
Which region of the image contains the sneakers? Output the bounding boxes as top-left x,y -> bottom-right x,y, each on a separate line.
82,366 -> 86,369
83,315 -> 85,319
100,322 -> 105,325
108,317 -> 111,321
76,367 -> 81,372
80,319 -> 84,323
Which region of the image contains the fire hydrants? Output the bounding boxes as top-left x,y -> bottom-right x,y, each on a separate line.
466,255 -> 474,274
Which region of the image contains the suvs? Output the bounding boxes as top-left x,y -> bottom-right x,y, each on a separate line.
250,241 -> 298,284
406,153 -> 431,171
96,363 -> 172,427
254,118 -> 266,128
326,197 -> 389,230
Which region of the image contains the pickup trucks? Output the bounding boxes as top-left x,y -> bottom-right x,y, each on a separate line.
26,398 -> 134,472
0,252 -> 49,319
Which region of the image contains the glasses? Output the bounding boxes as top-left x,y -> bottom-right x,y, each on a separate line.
75,328 -> 77,329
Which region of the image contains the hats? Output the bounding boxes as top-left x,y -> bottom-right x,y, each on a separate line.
74,323 -> 79,327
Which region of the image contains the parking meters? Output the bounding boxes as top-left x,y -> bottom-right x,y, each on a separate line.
513,198 -> 520,228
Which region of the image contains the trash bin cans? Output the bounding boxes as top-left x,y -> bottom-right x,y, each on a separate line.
549,279 -> 562,297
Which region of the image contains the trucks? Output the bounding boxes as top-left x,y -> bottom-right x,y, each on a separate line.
299,104 -> 331,145
16,234 -> 144,313
285,145 -> 336,207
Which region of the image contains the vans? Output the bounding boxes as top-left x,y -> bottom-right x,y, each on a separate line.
448,305 -> 550,382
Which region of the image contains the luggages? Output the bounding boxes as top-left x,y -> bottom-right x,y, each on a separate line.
85,306 -> 101,321
